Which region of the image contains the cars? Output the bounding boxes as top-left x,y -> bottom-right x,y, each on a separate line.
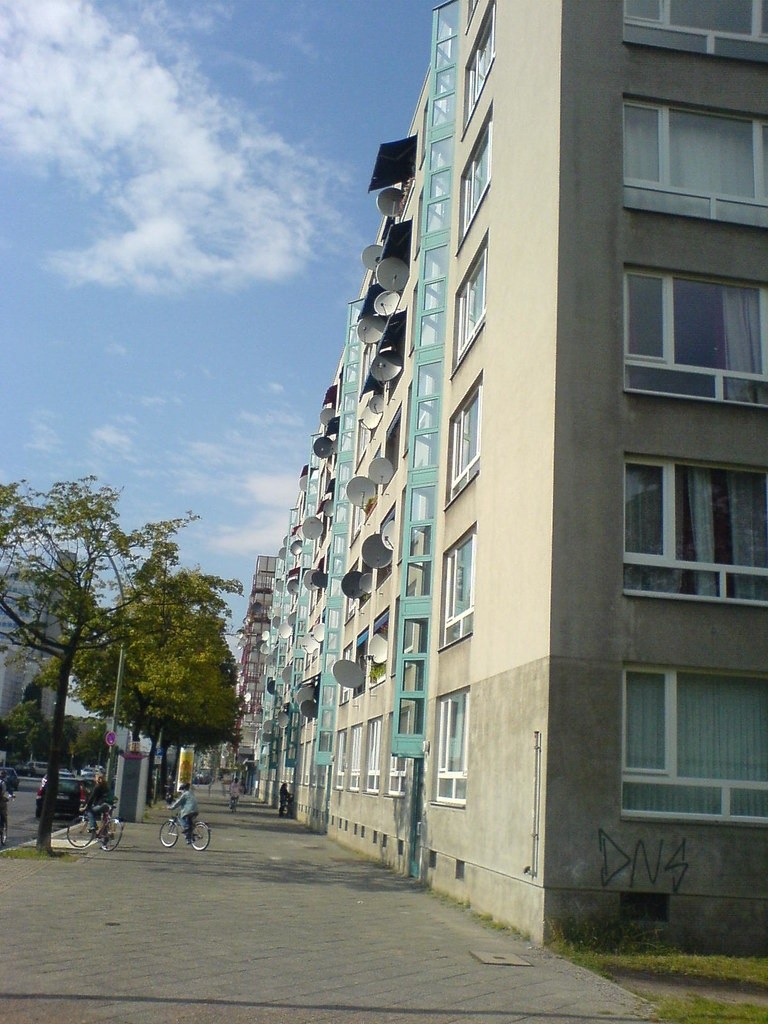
35,764 -> 104,819
0,768 -> 20,790
24,761 -> 47,777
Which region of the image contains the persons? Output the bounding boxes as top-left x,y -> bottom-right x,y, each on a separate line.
279,783 -> 292,818
229,778 -> 240,809
0,770 -> 13,836
78,776 -> 114,847
167,785 -> 198,844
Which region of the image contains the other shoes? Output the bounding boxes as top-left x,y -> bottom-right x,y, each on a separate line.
99,845 -> 108,849
181,828 -> 189,833
86,826 -> 98,833
279,814 -> 284,817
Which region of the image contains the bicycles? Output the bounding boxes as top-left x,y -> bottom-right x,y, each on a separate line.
230,794 -> 239,812
158,806 -> 211,851
0,796 -> 16,844
66,801 -> 125,852
